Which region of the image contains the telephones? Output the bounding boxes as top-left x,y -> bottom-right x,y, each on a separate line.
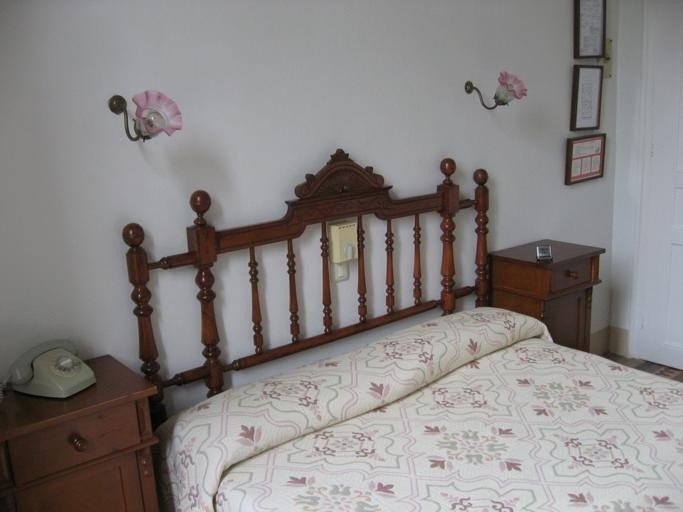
0,338 -> 96,399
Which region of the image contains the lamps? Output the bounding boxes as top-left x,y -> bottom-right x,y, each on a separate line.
108,90 -> 184,143
465,72 -> 528,110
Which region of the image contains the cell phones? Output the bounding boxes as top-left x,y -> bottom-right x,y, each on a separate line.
535,241 -> 553,259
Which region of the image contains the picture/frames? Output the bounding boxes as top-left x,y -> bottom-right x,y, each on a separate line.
570,64 -> 603,130
575,0 -> 606,58
565,133 -> 607,185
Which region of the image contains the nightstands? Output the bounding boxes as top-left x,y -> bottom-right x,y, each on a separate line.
0,355 -> 158,512
487,239 -> 606,353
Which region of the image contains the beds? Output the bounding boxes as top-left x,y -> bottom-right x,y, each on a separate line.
121,148 -> 683,512
